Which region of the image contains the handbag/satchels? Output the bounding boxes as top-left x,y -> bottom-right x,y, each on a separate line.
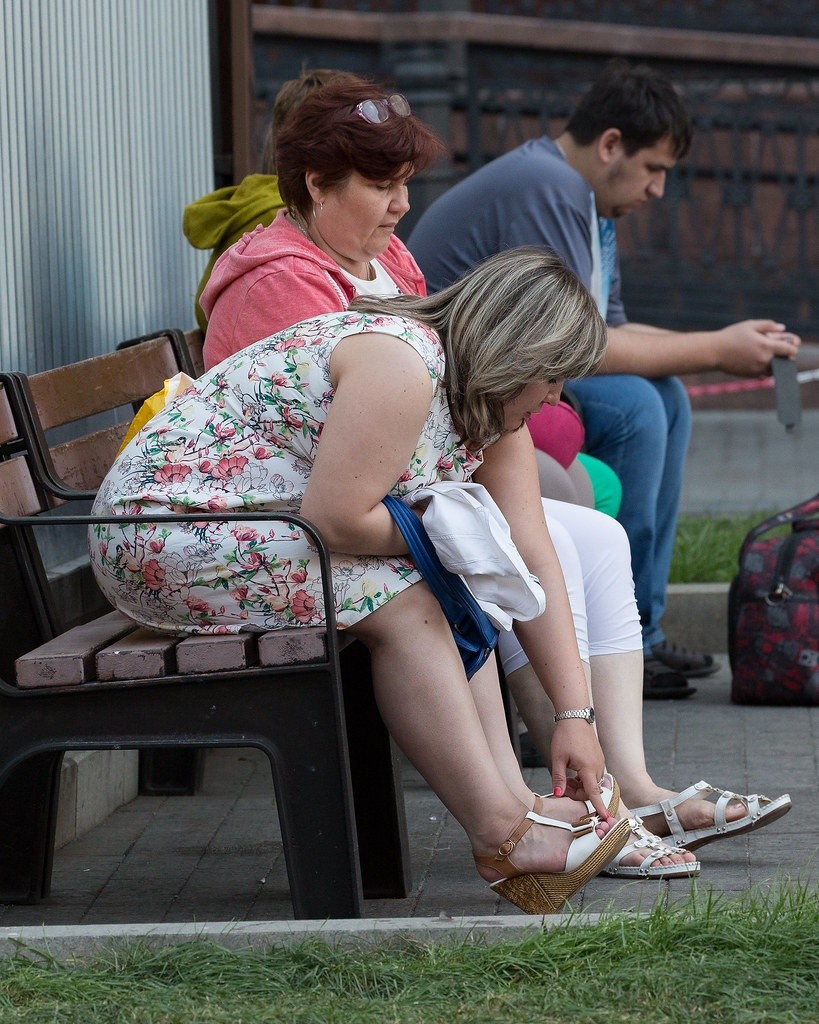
727,494 -> 819,706
381,494 -> 500,682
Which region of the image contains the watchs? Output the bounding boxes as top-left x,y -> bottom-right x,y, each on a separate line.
553,707 -> 595,725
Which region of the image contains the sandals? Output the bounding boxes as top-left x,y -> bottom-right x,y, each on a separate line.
597,814 -> 701,878
651,640 -> 722,676
643,656 -> 698,699
629,780 -> 792,853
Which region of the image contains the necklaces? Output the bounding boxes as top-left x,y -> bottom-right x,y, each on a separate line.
290,206 -> 376,280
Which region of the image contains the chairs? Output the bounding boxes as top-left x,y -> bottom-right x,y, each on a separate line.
0,326 -> 415,925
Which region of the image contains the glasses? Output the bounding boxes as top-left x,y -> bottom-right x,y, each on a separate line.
342,93 -> 412,125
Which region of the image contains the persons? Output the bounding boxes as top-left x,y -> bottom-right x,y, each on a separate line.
180,72 -> 797,877
87,243 -> 632,914
403,74 -> 799,698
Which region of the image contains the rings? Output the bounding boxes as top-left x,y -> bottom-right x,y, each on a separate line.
597,778 -> 604,785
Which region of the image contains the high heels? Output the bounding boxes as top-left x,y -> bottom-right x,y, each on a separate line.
472,773 -> 632,915
